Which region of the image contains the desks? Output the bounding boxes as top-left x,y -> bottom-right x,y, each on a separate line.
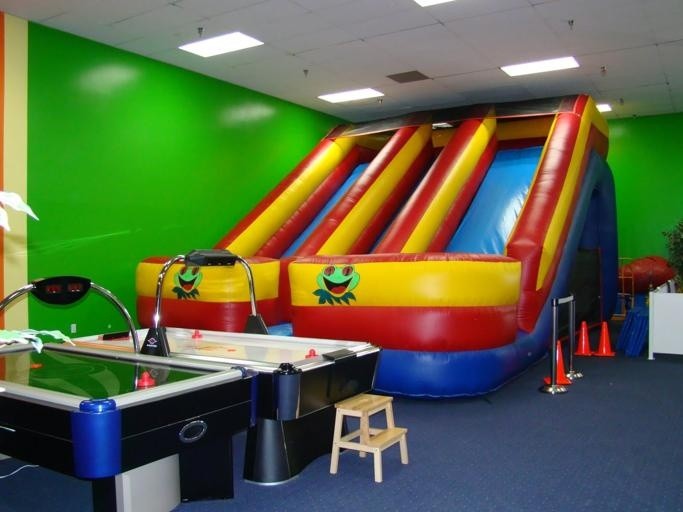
0,344 -> 259,512
72,326 -> 383,485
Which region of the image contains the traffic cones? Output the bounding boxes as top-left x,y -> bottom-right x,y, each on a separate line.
573,320 -> 593,355
592,319 -> 616,358
544,339 -> 572,385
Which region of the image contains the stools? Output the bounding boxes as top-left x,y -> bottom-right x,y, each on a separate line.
329,392 -> 409,483
